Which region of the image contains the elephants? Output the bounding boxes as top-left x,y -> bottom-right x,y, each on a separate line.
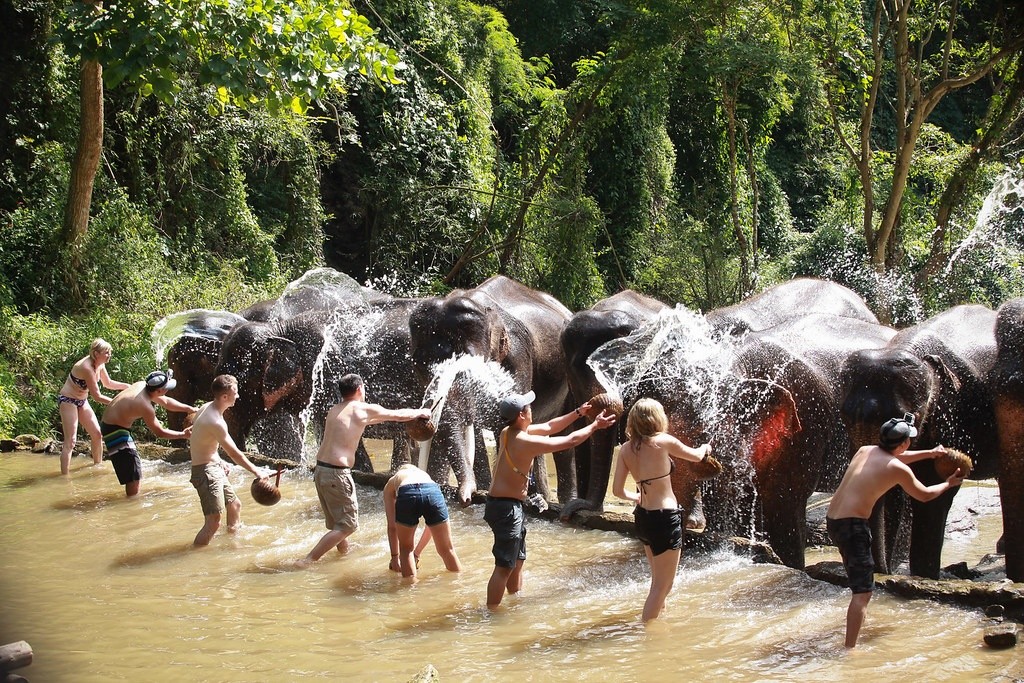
163,275 -> 1024,589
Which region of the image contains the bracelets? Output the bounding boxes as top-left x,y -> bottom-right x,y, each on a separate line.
575,407 -> 582,418
391,554 -> 398,556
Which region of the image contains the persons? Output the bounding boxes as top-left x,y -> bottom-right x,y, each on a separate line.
826,418 -> 966,648
482,389 -> 616,604
306,373 -> 431,561
190,375 -> 270,545
613,397 -> 712,623
57,338 -> 131,474
100,370 -> 199,496
383,464 -> 462,578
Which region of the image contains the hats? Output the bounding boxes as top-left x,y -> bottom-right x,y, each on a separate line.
500,390 -> 536,423
880,419 -> 918,447
145,371 -> 177,390
